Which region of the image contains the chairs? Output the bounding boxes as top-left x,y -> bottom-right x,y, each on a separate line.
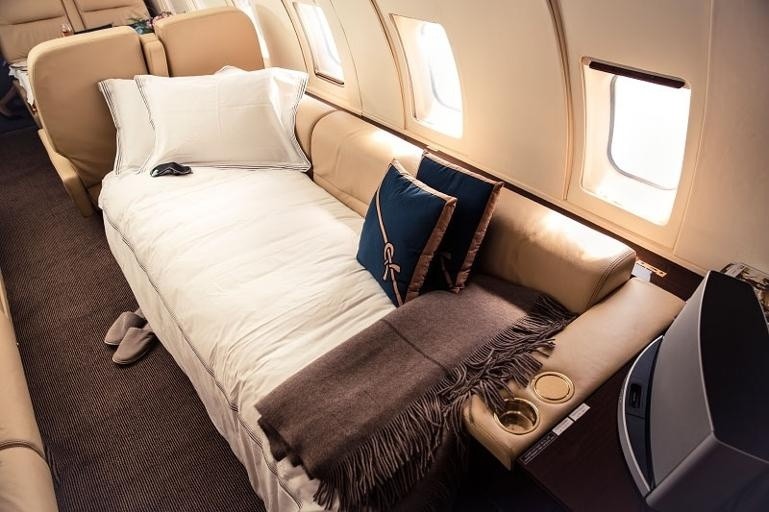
0,0 -> 265,218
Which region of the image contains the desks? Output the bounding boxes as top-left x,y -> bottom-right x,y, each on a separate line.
514,350 -> 652,512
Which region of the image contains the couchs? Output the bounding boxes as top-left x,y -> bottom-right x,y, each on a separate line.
98,93 -> 686,511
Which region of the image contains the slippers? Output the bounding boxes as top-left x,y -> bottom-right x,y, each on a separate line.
103,306 -> 155,365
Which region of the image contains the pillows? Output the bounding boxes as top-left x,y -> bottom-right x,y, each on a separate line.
355,150 -> 504,308
97,66 -> 311,174
